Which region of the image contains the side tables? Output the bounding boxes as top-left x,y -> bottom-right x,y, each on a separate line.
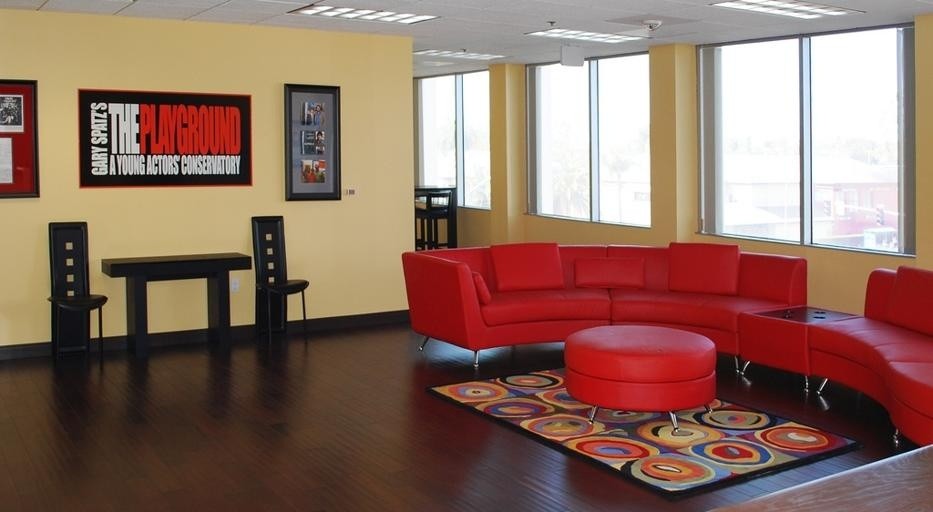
738,305 -> 864,392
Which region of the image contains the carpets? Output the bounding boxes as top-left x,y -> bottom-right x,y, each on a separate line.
424,366 -> 866,501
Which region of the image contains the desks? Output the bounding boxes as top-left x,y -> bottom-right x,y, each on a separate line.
101,252 -> 251,355
415,188 -> 457,250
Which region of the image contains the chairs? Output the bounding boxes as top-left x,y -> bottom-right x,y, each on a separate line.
48,222 -> 107,374
252,216 -> 309,357
416,190 -> 452,250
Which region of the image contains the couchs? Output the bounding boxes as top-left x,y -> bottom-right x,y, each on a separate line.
808,265 -> 932,446
403,243 -> 808,373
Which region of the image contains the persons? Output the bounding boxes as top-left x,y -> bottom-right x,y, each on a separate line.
302,164 -> 324,183
312,104 -> 325,126
316,131 -> 324,140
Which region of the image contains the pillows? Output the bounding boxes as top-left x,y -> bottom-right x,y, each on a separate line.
668,242 -> 741,297
574,257 -> 644,289
490,243 -> 566,291
471,271 -> 491,305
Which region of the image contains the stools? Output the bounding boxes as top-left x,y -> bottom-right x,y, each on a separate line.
564,325 -> 717,434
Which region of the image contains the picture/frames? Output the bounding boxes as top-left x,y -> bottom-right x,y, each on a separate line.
0,80 -> 40,197
284,83 -> 341,200
